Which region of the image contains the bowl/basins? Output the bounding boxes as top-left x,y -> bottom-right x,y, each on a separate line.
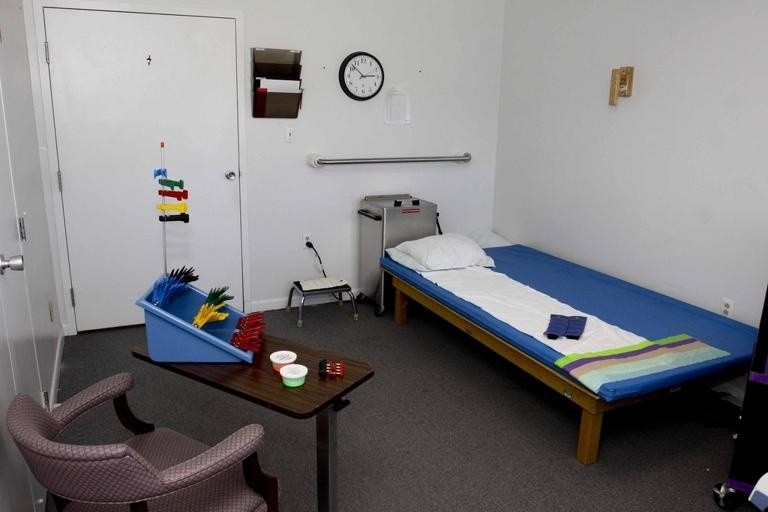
269,350 -> 309,387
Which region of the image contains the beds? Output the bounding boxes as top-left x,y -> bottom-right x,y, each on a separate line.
378,243 -> 760,464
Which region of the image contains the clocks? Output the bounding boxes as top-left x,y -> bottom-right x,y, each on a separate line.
339,52 -> 385,101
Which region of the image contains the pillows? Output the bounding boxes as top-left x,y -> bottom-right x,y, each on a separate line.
397,234 -> 496,269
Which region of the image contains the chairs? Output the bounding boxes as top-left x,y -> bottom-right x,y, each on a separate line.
6,374 -> 291,512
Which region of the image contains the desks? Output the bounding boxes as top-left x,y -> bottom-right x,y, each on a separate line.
131,330 -> 376,512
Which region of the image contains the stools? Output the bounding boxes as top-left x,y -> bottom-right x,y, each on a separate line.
285,280 -> 358,328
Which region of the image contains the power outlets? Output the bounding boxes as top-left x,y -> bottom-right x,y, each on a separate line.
303,240 -> 313,250
